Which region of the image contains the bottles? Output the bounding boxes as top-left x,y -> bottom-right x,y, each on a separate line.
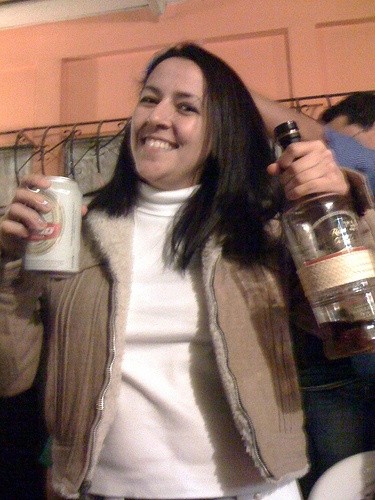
272,119 -> 375,361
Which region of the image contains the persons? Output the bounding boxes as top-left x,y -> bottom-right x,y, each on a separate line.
246,88 -> 375,393
2,40 -> 374,500
